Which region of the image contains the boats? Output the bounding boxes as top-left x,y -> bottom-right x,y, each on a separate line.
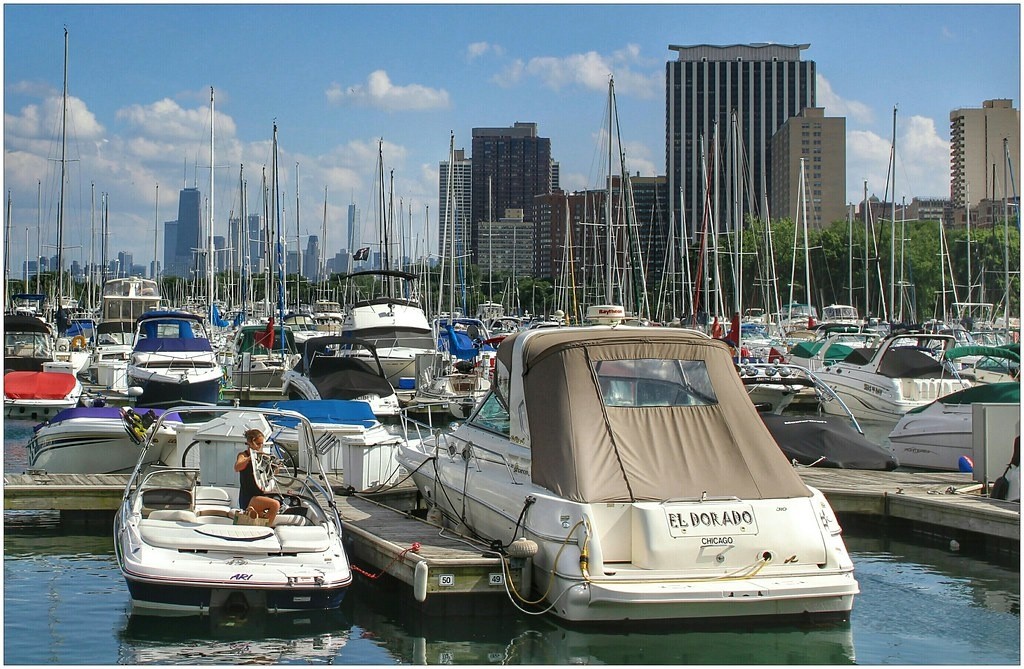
390,301 -> 860,624
109,396 -> 355,617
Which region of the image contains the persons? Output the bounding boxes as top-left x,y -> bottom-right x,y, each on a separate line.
234,430 -> 280,527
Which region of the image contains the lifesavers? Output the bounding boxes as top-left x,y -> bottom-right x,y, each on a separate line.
72,335 -> 86,348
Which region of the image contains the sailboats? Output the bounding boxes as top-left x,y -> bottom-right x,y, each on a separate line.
4,20 -> 1021,493
